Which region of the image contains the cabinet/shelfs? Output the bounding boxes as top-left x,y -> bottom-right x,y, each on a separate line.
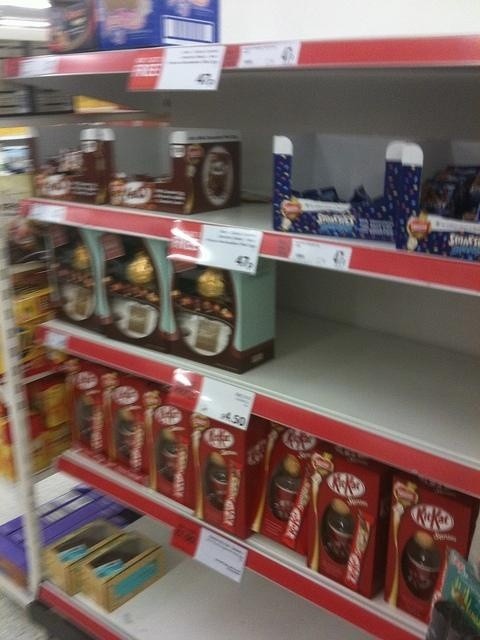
0,33 -> 480,639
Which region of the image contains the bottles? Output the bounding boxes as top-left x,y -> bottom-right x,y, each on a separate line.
75,395 -> 441,597
